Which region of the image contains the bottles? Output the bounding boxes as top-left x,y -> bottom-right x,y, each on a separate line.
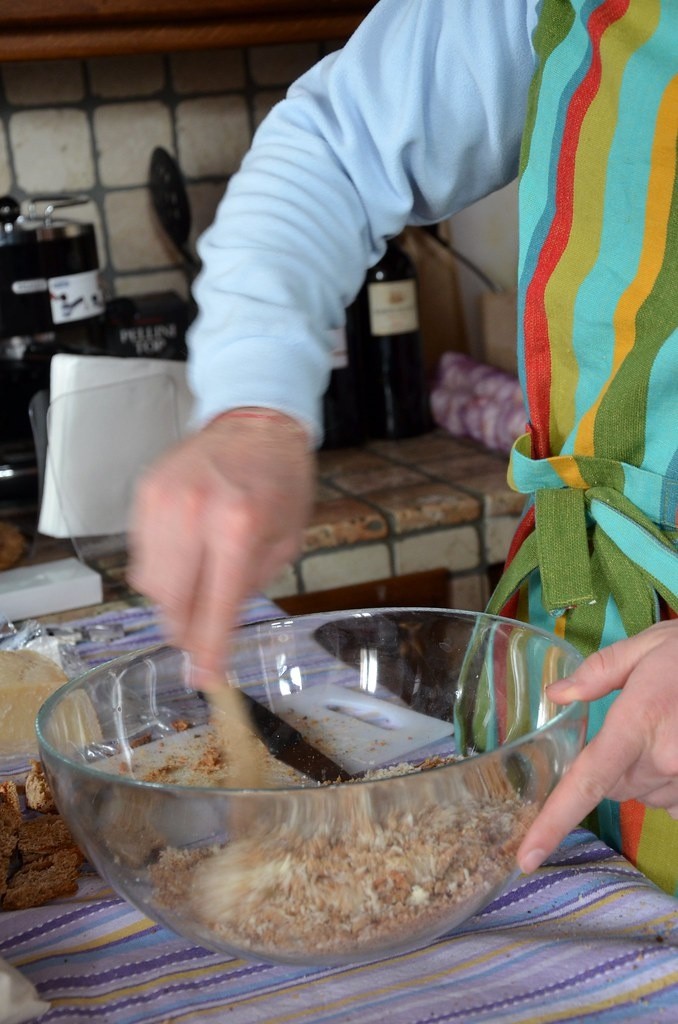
357,238 -> 432,439
322,308 -> 377,447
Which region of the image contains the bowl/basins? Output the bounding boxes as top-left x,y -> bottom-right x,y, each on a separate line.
35,607 -> 589,966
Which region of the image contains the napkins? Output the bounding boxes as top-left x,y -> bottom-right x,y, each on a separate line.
37,353 -> 198,539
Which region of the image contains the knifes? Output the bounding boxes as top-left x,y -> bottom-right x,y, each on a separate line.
191,674 -> 353,785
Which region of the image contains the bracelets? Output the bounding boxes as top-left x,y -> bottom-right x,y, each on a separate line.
199,407 -> 309,455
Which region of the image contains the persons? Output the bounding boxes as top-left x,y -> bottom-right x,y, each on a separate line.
125,0 -> 678,897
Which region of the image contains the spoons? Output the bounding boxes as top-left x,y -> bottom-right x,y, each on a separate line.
150,145 -> 206,273
